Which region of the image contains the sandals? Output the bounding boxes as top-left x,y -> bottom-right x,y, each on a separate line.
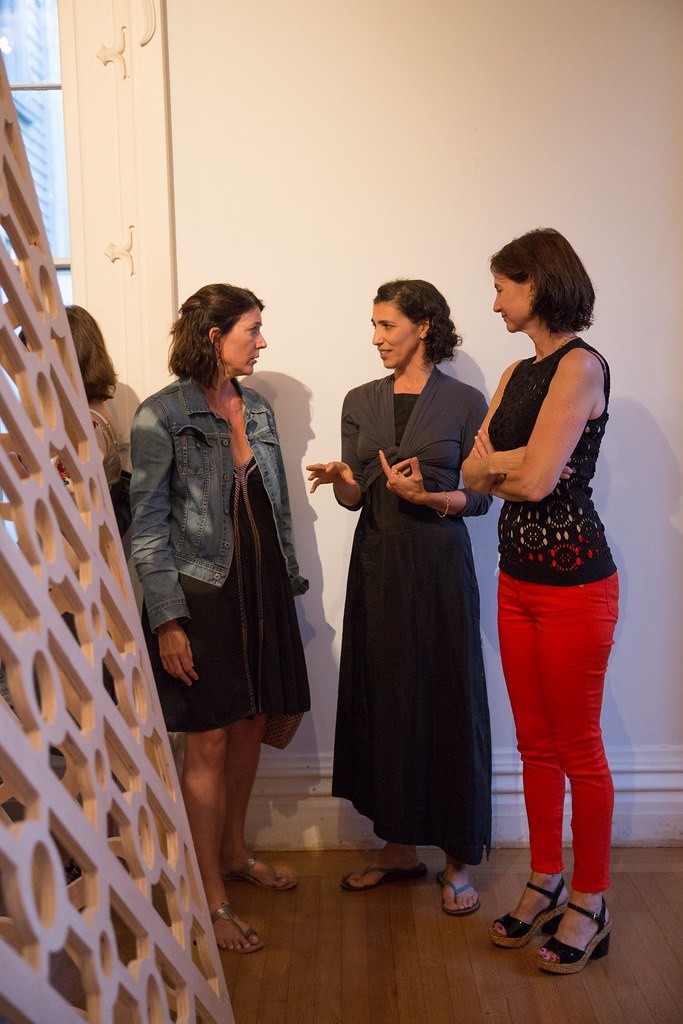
222,854 -> 297,890
210,904 -> 264,953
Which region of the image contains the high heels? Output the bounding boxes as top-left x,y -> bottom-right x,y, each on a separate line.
489,877 -> 568,948
535,895 -> 612,974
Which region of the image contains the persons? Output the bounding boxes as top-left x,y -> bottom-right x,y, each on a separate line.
8,305 -> 125,887
460,229 -> 621,975
307,279 -> 492,916
129,285 -> 310,952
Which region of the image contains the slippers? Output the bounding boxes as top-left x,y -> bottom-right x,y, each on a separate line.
437,871 -> 480,915
340,861 -> 428,892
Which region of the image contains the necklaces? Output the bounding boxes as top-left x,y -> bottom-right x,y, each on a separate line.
211,400 -> 233,433
559,335 -> 578,348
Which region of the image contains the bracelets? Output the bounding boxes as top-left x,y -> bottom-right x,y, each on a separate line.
438,491 -> 450,518
